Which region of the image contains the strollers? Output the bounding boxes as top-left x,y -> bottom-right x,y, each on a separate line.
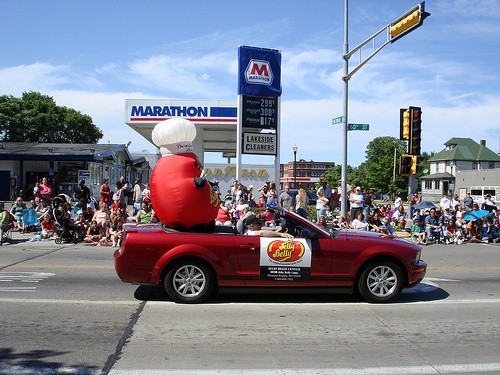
52,206 -> 85,245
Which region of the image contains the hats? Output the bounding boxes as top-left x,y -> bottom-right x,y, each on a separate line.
356,186 -> 361,191
245,215 -> 265,225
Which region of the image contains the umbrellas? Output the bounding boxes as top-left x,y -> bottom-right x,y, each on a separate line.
463,210 -> 489,220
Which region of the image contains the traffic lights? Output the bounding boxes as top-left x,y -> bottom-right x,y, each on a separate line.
412,156 -> 425,174
399,156 -> 412,176
408,106 -> 422,155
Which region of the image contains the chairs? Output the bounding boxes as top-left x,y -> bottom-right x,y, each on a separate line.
3,212 -> 17,243
420,221 -> 440,244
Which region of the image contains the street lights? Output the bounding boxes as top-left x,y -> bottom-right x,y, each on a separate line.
292,143 -> 299,189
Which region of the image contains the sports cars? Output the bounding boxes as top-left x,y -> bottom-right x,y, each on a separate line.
113,205 -> 429,303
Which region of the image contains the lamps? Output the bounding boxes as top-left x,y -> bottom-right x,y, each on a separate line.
0,145 -> 3,149
48,148 -> 52,153
91,148 -> 95,153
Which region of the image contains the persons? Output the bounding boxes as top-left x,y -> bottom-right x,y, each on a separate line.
352,192 -> 500,245
127,178 -> 155,223
0,177 -> 52,246
52,178 -> 95,242
259,180 -> 364,228
242,211 -> 294,242
215,179 -> 254,225
84,175 -> 129,247
